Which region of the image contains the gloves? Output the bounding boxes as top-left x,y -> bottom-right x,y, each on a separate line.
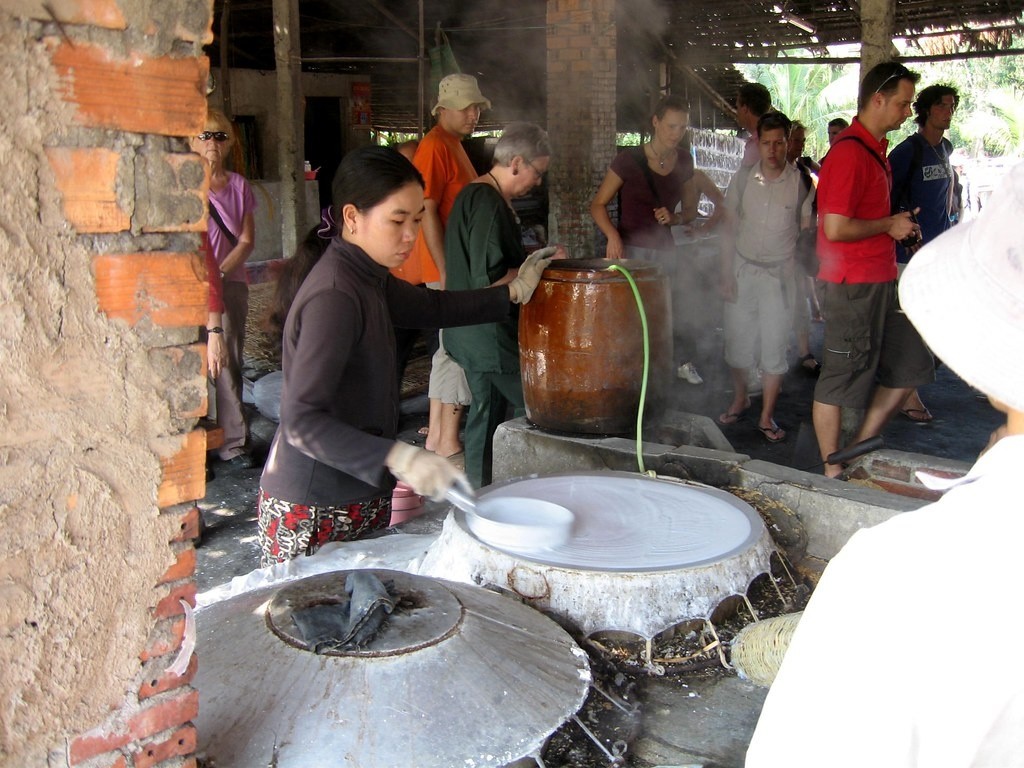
509,246 -> 558,306
390,443 -> 476,502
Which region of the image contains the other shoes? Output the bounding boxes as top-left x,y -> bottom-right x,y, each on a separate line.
811,310 -> 823,322
415,422 -> 430,437
825,462 -> 849,481
444,443 -> 467,468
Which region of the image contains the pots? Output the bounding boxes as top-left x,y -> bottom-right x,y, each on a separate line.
305,166 -> 322,180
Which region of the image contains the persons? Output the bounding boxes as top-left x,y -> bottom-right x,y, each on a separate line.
188,107 -> 255,469
387,73 -> 492,471
443,121 -> 553,491
590,94 -> 698,290
743,160 -> 1024,768
813,61 -> 934,479
256,143 -> 558,568
672,81 -> 983,442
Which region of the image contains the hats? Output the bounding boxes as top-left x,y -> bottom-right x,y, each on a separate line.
430,73 -> 493,116
896,156 -> 1024,412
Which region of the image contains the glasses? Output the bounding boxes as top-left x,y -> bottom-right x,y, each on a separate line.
528,160 -> 545,180
876,69 -> 916,95
197,131 -> 231,141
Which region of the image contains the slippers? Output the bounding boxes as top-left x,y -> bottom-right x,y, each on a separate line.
714,409 -> 753,426
757,424 -> 786,442
899,406 -> 934,422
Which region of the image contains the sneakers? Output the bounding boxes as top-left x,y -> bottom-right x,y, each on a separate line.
677,362 -> 704,384
227,453 -> 253,467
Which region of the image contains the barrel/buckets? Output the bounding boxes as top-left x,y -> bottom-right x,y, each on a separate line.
518,256 -> 672,436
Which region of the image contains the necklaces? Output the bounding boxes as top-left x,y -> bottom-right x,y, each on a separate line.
650,140 -> 672,168
487,172 -> 521,225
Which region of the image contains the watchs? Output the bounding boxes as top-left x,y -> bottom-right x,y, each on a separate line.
220,271 -> 226,280
207,327 -> 224,333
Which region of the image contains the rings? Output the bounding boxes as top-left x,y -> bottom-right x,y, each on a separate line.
222,363 -> 226,365
661,217 -> 665,221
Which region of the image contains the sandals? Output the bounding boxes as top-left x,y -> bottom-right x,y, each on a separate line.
794,354 -> 823,377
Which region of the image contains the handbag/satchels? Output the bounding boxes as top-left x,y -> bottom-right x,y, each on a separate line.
793,224 -> 820,278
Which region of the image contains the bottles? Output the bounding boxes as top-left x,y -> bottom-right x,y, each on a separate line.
304,161 -> 312,172
